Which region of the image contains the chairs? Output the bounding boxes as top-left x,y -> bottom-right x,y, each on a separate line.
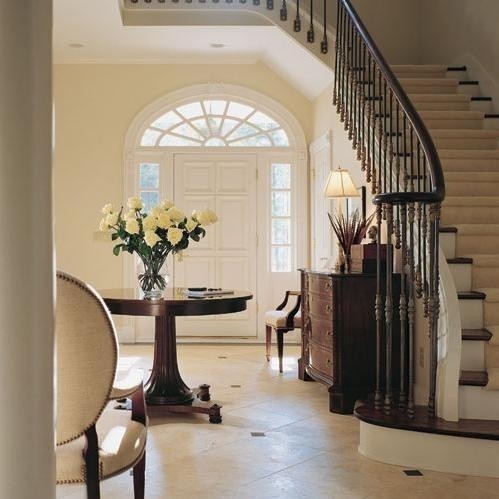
55,270 -> 147,498
264,289 -> 302,375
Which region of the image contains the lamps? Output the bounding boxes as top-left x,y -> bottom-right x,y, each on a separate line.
323,166 -> 360,200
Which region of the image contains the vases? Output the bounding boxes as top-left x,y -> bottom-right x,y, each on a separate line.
133,258 -> 168,301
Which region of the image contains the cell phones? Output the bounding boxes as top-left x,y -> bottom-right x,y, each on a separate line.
188,287 -> 208,291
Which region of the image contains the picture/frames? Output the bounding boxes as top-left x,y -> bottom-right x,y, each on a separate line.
344,185 -> 367,229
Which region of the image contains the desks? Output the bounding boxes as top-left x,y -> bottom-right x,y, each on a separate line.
100,289 -> 254,426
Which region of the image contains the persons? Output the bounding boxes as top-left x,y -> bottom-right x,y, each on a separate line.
367,225 -> 377,244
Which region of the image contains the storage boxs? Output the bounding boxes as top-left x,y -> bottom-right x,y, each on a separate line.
347,243 -> 392,275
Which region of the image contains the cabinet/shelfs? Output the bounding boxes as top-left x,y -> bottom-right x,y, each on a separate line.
296,266 -> 408,418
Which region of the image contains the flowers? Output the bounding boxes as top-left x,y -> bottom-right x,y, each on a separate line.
96,194 -> 218,289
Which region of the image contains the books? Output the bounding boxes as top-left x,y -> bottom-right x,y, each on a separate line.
180,288 -> 235,296
